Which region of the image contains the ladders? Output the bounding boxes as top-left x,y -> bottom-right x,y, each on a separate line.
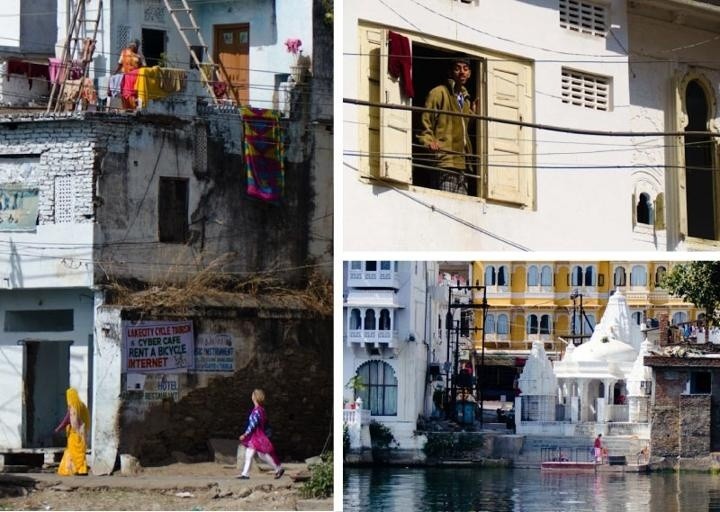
164,0 -> 238,109
54,0 -> 103,112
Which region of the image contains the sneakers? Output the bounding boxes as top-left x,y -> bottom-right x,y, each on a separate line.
235,473 -> 250,480
273,468 -> 285,479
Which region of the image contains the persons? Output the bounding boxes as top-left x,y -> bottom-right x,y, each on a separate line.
593,433 -> 603,461
666,320 -> 720,344
111,38 -> 147,75
418,59 -> 479,196
235,389 -> 285,483
53,387 -> 91,474
636,446 -> 650,465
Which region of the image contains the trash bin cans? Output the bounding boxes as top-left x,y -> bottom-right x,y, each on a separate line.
456,400 -> 476,425
